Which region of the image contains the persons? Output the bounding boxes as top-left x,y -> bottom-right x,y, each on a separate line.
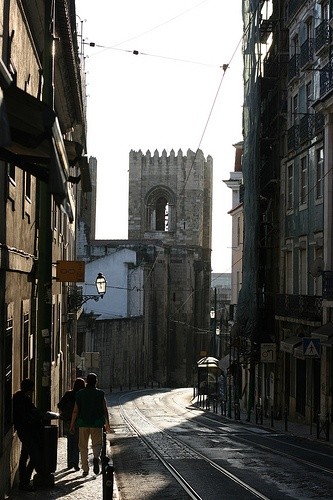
58,378 -> 86,470
13,380 -> 52,491
70,373 -> 111,476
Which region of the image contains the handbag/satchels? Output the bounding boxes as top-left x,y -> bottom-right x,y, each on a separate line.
61,409 -> 71,432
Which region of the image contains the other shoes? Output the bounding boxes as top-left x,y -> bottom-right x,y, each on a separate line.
83,472 -> 88,475
93,458 -> 99,474
74,464 -> 80,471
19,482 -> 35,491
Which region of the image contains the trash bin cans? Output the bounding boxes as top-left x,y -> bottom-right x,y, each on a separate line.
32,425 -> 58,488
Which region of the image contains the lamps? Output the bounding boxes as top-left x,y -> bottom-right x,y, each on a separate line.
68,272 -> 107,311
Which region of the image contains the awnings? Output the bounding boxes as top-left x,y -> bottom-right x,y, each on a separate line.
219,322 -> 333,372
7,83 -> 74,223
